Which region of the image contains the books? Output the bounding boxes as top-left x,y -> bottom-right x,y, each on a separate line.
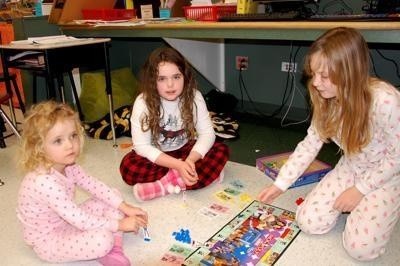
9,35 -> 93,46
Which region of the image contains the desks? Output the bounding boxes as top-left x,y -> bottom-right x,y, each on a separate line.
0,35 -> 118,148
55,18 -> 400,44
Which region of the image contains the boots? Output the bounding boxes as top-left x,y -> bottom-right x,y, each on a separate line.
97,246 -> 131,266
132,169 -> 186,202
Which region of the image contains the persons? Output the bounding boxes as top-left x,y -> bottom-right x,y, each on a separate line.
255,27 -> 400,261
119,48 -> 230,202
15,100 -> 148,266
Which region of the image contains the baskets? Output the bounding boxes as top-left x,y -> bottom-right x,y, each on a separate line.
80,7 -> 137,21
181,4 -> 237,21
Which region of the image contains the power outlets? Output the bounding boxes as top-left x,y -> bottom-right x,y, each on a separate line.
281,62 -> 297,73
235,56 -> 248,71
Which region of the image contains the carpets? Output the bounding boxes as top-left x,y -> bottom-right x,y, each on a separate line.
1,103 -> 400,266
